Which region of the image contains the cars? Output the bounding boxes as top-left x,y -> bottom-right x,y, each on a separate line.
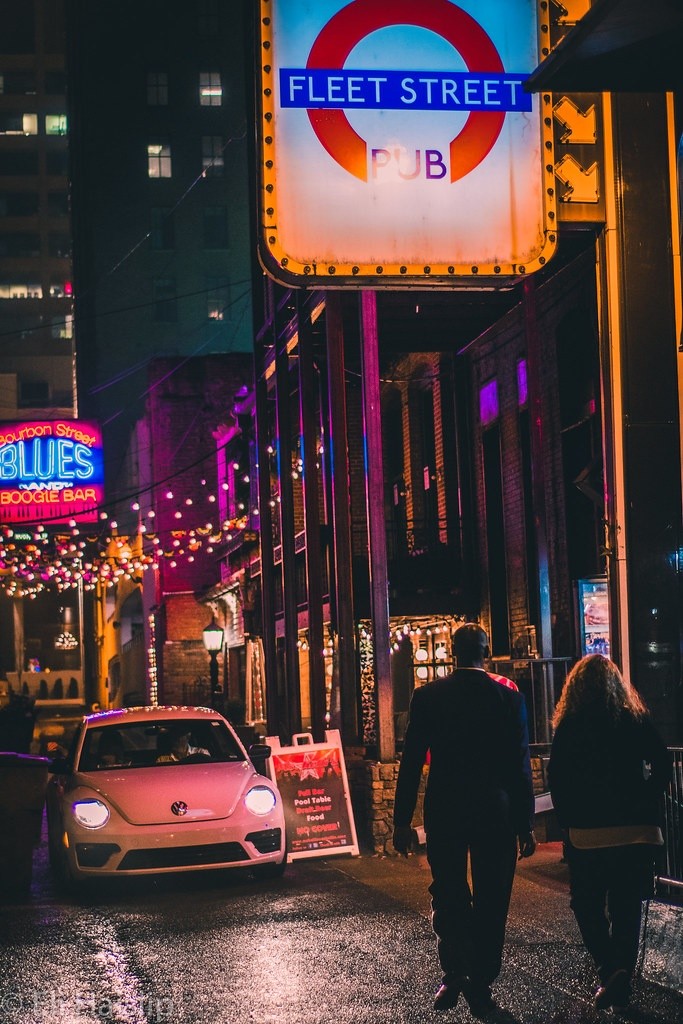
45,706 -> 288,903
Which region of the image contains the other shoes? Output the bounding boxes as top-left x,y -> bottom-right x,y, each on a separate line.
594,959 -> 629,1008
612,1000 -> 629,1014
432,972 -> 497,1014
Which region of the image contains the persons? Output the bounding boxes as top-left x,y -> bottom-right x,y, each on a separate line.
396,623 -> 538,1002
48,742 -> 133,765
157,729 -> 210,762
89,700 -> 101,712
551,654 -> 673,1009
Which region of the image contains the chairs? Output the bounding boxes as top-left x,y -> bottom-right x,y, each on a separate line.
157,733 -> 194,749
99,730 -> 124,753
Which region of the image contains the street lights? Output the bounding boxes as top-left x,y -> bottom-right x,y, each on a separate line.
202,615 -> 224,710
